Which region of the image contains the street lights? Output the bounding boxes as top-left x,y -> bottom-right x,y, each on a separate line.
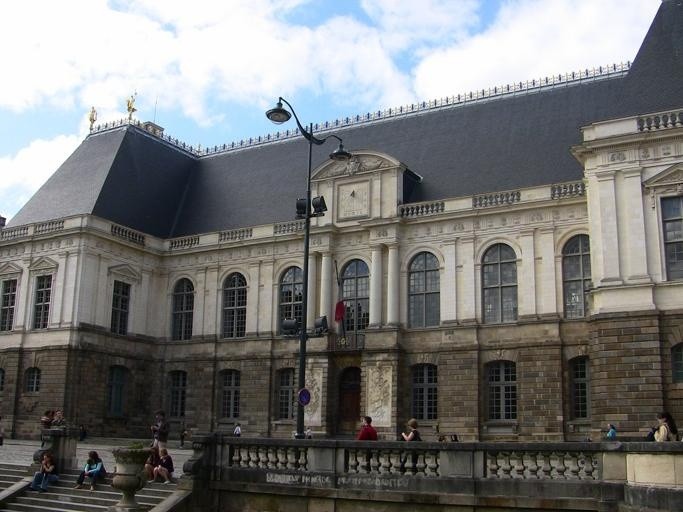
265,96 -> 351,469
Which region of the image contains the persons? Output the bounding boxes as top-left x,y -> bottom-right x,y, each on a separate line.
357,416 -> 377,440
233,423 -> 242,435
305,427 -> 312,438
606,423 -> 616,441
401,418 -> 420,441
74,451 -> 106,491
28,453 -> 58,492
653,412 -> 677,442
40,410 -> 65,448
145,409 -> 175,484
